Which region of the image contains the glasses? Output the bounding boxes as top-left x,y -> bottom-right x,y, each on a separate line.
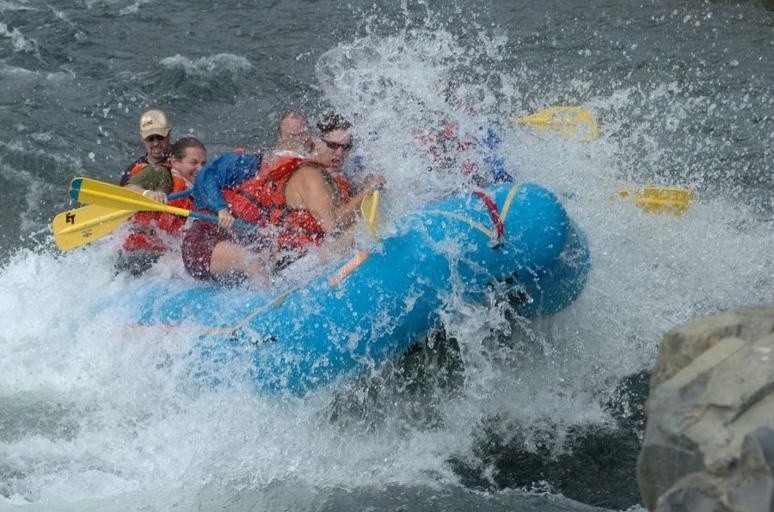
320,138 -> 353,153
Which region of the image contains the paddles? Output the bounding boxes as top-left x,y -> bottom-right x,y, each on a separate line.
52,188 -> 190,251
360,183 -> 383,241
605,186 -> 690,215
68,177 -> 258,232
508,105 -> 596,142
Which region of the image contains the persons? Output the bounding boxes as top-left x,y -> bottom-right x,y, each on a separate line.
183,113 -> 384,290
193,114 -> 313,229
116,138 -> 207,276
117,110 -> 172,186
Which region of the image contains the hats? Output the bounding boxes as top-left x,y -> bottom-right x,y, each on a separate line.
139,110 -> 171,139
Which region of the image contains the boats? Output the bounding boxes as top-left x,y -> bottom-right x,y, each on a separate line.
63,182 -> 593,401
